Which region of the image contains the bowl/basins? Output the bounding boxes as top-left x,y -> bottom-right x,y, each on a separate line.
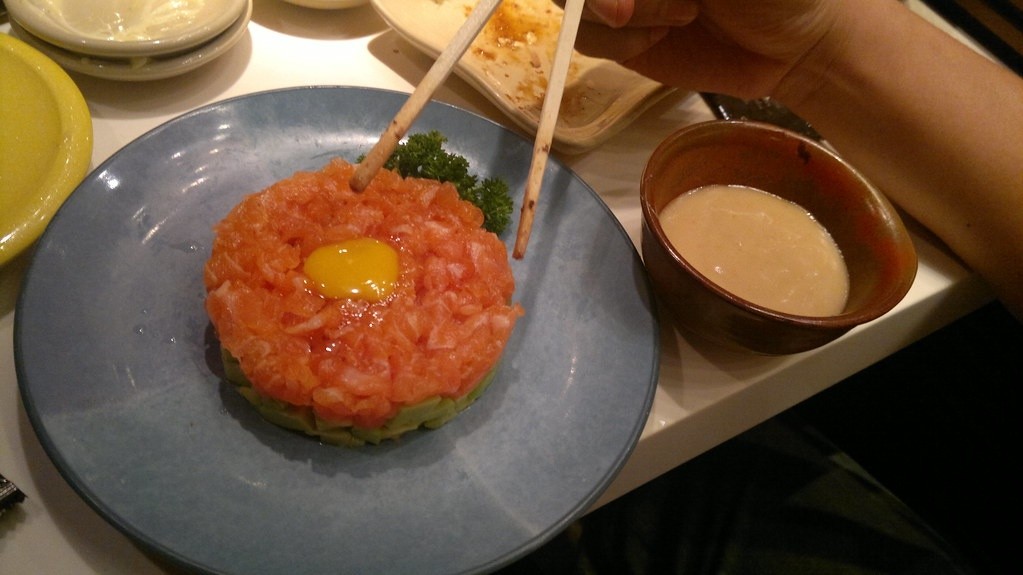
637,119 -> 920,357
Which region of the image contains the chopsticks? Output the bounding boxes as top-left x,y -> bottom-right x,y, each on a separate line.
348,1 -> 586,262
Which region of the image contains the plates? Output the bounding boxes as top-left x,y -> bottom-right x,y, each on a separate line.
0,32 -> 94,268
12,84 -> 662,575
371,0 -> 679,157
3,1 -> 253,82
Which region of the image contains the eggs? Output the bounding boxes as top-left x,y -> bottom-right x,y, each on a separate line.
302,235 -> 401,305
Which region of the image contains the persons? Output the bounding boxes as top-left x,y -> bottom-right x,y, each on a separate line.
551,0 -> 1023,315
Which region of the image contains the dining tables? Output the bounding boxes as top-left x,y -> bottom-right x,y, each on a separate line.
0,0 -> 1023,574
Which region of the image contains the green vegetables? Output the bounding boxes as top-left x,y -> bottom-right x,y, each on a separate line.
357,129 -> 515,238
